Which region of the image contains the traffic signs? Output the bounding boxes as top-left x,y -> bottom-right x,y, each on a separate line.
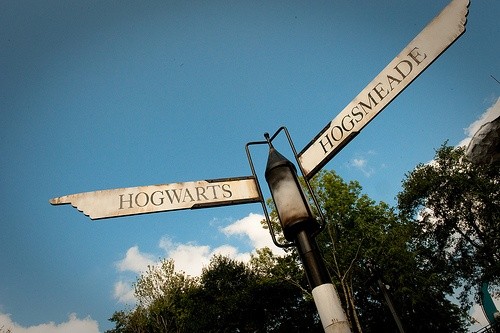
50,176 -> 260,221
298,0 -> 470,180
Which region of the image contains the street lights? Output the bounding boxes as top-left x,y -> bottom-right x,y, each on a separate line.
248,126 -> 354,332
